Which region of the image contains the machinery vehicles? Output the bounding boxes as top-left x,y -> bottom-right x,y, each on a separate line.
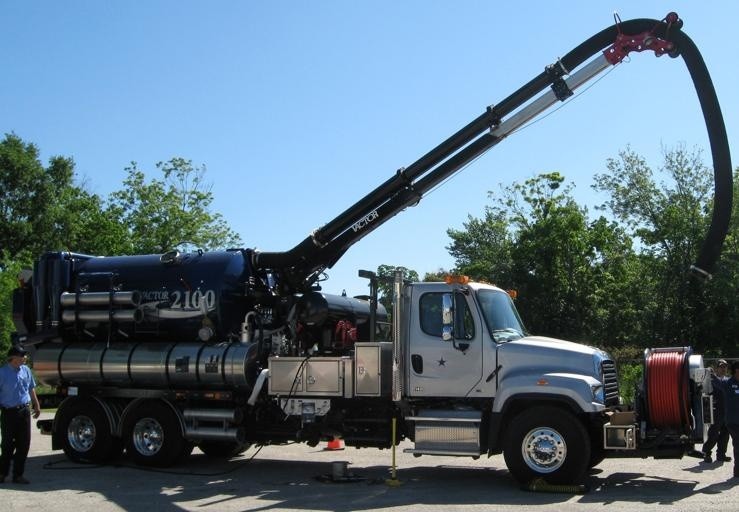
6,9 -> 735,492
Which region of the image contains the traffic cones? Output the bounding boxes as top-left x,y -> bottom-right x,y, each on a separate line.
323,436 -> 346,451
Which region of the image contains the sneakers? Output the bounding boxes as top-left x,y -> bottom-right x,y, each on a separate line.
702,447 -> 731,463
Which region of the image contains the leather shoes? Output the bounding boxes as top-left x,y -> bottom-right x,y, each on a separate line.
12,476 -> 31,484
0,474 -> 6,483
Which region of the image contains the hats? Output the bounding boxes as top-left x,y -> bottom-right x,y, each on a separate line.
7,346 -> 30,357
717,359 -> 728,367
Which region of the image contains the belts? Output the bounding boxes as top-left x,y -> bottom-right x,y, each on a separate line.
10,403 -> 29,410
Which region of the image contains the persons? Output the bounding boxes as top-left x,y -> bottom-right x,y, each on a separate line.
711,359 -> 739,480
703,359 -> 732,463
0,347 -> 40,484
